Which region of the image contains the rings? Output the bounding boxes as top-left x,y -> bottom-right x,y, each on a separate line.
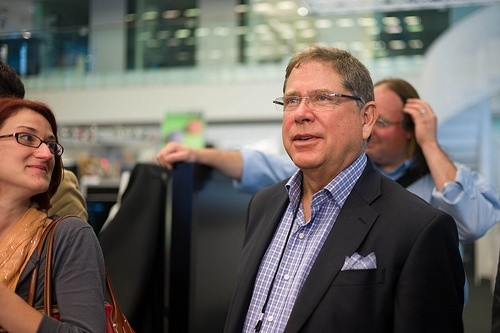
421,108 -> 427,113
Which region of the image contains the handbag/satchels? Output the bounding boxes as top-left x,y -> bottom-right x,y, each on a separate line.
29,213 -> 135,332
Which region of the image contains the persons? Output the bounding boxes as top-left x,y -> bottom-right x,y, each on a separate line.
224,45 -> 465,333
156,78 -> 500,300
0,59 -> 88,223
0,98 -> 106,333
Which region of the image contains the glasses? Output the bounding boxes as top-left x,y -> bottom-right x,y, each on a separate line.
0,131 -> 64,157
273,92 -> 364,109
